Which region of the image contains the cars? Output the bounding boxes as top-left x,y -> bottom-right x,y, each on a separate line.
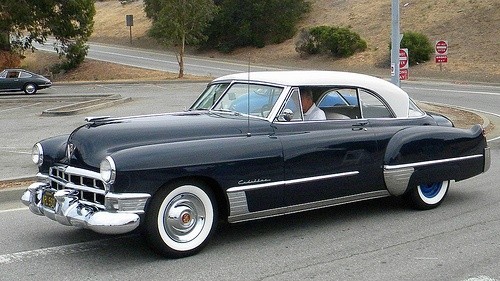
20,71 -> 491,259
0,69 -> 52,95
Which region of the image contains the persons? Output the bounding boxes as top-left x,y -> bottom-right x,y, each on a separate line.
9,73 -> 18,78
53,37 -> 59,54
290,87 -> 326,121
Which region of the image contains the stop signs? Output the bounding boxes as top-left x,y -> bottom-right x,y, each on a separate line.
400,49 -> 409,69
435,40 -> 448,55
